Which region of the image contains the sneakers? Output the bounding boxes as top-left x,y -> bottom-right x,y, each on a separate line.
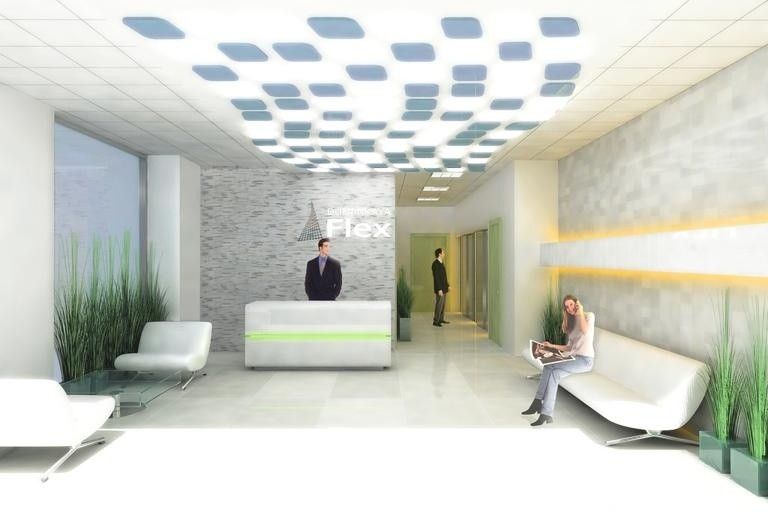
432,320 -> 451,328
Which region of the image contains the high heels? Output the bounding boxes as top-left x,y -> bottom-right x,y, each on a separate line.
521,399 -> 544,415
530,413 -> 554,426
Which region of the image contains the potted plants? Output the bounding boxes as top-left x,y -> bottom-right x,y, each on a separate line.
107,227 -> 127,390
56,235 -> 93,397
87,244 -> 109,394
730,288 -> 768,499
396,260 -> 415,343
692,272 -> 748,475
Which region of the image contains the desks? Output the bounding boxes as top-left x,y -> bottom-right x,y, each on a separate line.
63,368 -> 182,421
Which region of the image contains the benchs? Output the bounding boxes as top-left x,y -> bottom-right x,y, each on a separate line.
522,316 -> 711,451
0,371 -> 119,484
113,319 -> 217,392
